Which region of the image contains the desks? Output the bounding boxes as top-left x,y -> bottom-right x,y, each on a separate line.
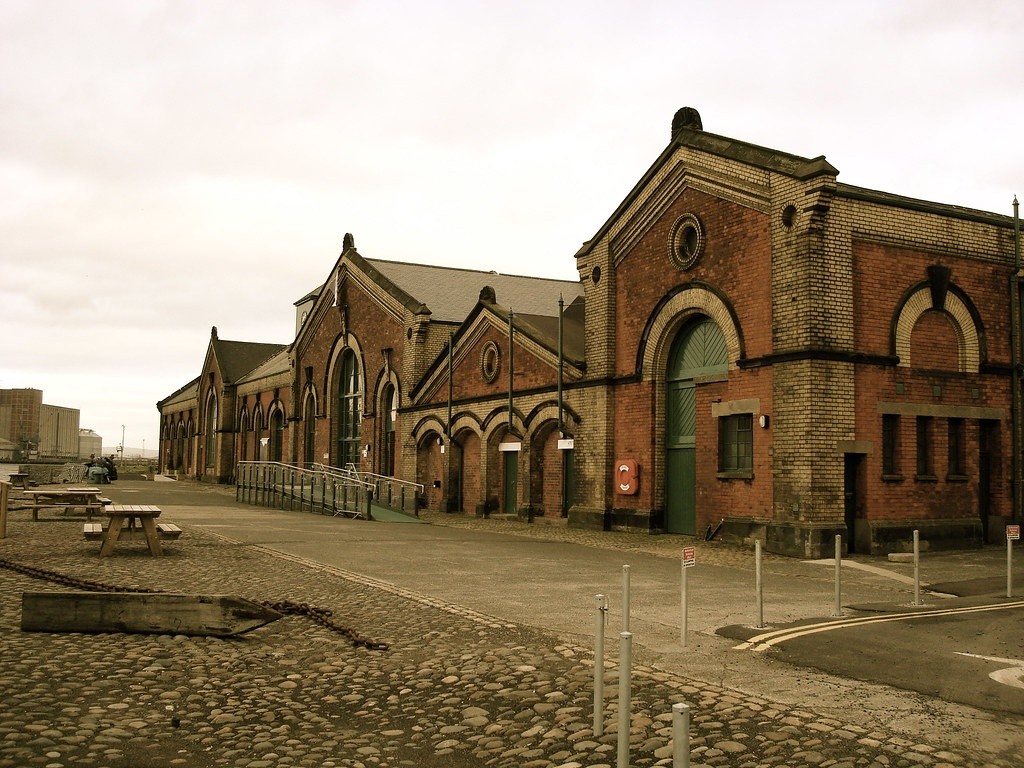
8,474 -> 30,490
23,490 -> 102,521
66,486 -> 99,503
99,504 -> 166,559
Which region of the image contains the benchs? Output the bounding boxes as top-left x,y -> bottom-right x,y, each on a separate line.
23,496 -> 112,522
157,523 -> 182,540
83,523 -> 103,542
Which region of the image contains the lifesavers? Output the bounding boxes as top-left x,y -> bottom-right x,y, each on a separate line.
616,464 -> 631,492
